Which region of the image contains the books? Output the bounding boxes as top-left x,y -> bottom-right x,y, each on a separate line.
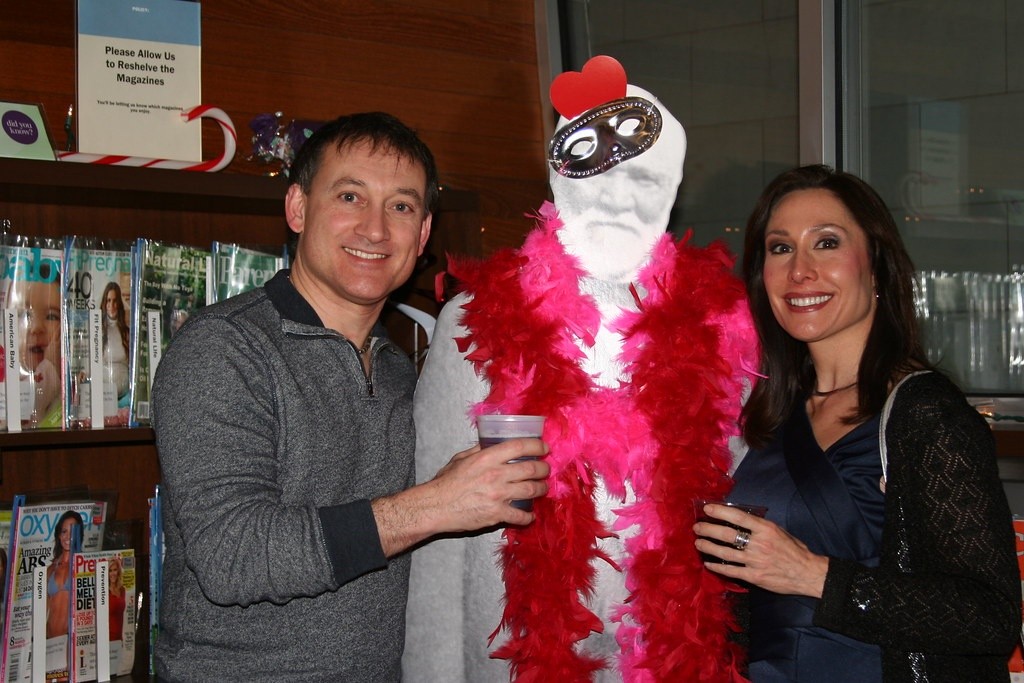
0,232 -> 284,432
0,483 -> 167,683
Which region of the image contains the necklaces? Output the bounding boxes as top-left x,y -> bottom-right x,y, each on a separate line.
446,201 -> 762,683
815,382 -> 857,395
359,332 -> 374,353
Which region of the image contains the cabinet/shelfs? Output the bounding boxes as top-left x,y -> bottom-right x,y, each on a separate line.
0,156 -> 485,683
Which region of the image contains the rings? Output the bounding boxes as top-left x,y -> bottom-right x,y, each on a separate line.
735,530 -> 750,548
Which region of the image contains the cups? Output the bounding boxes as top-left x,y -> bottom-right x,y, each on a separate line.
475,412 -> 546,514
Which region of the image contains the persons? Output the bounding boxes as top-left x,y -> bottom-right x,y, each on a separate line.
6,264 -> 60,427
152,113 -> 552,683
100,282 -> 130,399
692,163 -> 1024,683
108,561 -> 125,641
46,511 -> 84,640
399,83 -> 765,683
